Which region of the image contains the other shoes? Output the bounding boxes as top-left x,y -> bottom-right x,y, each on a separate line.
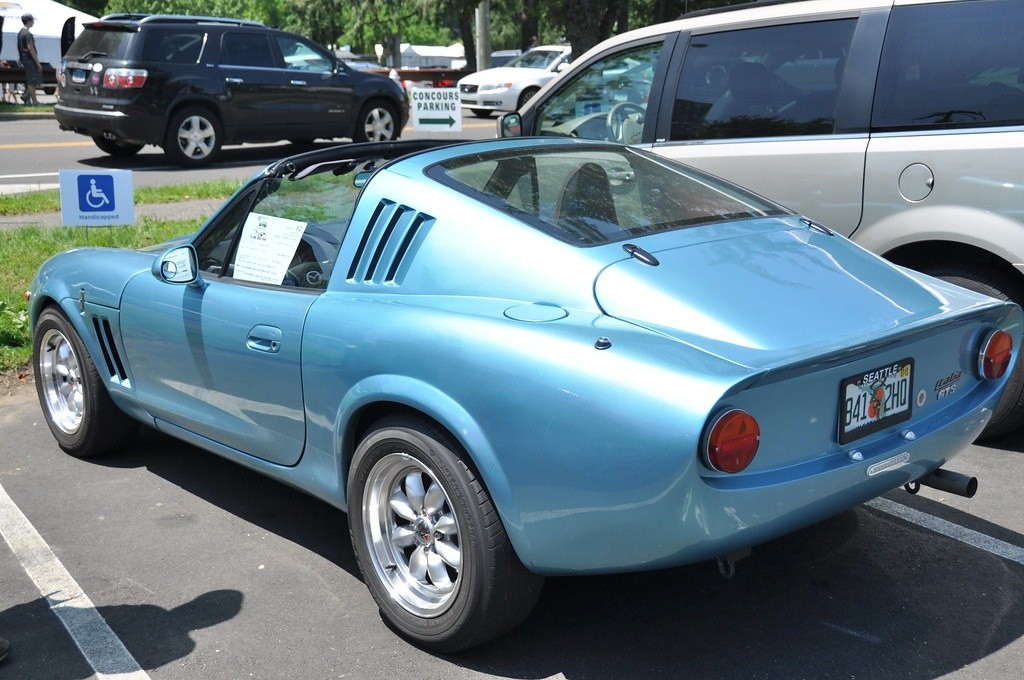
21,95 -> 47,107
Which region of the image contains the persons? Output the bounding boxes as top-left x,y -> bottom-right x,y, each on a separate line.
16,12 -> 45,109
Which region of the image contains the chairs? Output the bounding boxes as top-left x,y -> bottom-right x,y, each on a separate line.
552,161 -> 619,237
703,61 -> 769,134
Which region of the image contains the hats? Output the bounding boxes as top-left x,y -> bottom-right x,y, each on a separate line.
21,14 -> 38,21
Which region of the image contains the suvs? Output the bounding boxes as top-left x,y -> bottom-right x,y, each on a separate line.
54,14 -> 411,170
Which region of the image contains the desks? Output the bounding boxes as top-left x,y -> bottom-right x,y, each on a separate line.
0,68 -> 57,103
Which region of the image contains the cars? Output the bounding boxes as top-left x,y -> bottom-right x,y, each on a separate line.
456,45 -> 653,120
26,136 -> 1023,654
436,50 -> 523,88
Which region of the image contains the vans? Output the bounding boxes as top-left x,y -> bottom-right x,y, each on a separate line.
443,0 -> 1024,447
